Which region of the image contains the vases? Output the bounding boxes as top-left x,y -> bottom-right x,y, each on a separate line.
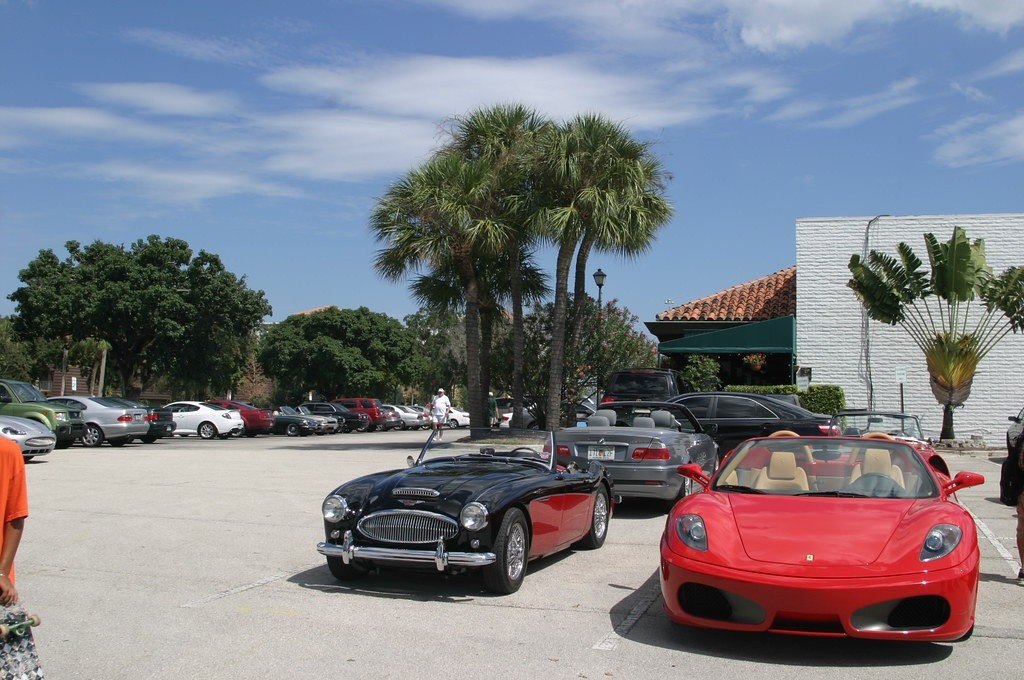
751,364 -> 761,369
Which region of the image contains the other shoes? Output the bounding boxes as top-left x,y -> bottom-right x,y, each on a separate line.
432,436 -> 443,441
1016,568 -> 1024,586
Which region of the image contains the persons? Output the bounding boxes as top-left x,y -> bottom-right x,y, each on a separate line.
1013,427 -> 1024,581
0,434 -> 29,607
430,388 -> 450,441
488,392 -> 499,428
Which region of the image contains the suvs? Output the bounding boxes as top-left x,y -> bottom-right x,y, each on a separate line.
1,378 -> 87,450
331,398 -> 387,432
496,397 -> 531,423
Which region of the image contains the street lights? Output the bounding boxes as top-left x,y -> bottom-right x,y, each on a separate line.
593,268 -> 608,409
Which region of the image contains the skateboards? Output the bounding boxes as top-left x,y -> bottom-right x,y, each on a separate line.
0,601 -> 45,680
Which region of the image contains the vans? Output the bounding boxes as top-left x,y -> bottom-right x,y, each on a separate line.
601,370 -> 695,416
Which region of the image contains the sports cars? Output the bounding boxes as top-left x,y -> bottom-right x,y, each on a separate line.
0,415 -> 56,462
658,430 -> 986,644
316,425 -> 624,595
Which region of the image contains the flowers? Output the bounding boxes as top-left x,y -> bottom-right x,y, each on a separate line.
742,352 -> 766,366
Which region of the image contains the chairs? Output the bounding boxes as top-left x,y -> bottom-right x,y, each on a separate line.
633,417 -> 655,428
595,410 -> 617,427
849,449 -> 905,490
650,411 -> 675,429
587,416 -> 610,427
754,452 -> 809,491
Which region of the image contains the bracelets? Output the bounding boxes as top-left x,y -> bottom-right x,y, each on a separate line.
0,574 -> 8,576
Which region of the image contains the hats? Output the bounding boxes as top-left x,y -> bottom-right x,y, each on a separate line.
438,388 -> 445,392
489,392 -> 494,396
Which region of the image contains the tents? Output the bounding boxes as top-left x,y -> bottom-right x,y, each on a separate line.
658,314 -> 796,385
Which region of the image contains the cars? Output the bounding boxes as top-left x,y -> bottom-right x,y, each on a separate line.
445,407 -> 471,428
617,391 -> 842,463
542,400 -> 722,505
46,396 -> 177,448
376,404 -> 440,431
499,400 -> 597,431
822,411 -> 934,446
180,401 -> 275,440
162,401 -> 245,440
271,406 -> 347,436
